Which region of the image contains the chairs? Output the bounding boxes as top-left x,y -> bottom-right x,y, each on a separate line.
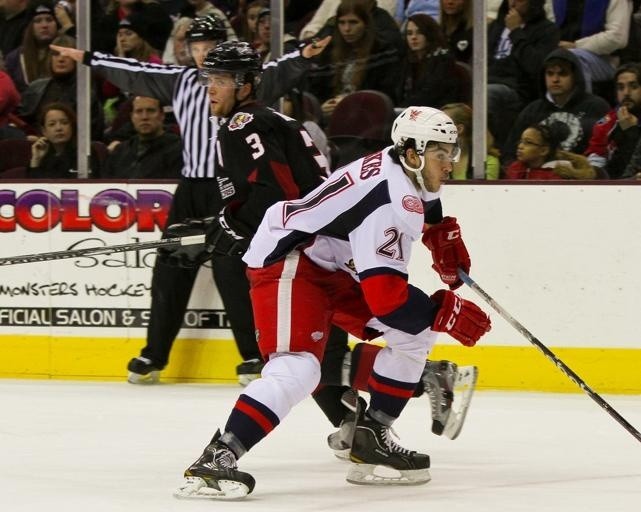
321,90 -> 400,173
0,91 -> 181,180
454,62 -> 474,105
283,83 -> 321,127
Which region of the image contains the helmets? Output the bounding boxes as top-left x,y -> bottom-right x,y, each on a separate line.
198,41 -> 263,87
184,16 -> 225,41
390,105 -> 462,164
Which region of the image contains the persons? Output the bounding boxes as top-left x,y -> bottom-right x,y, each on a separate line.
166,40 -> 458,452
182,105 -> 493,495
48,13 -> 333,376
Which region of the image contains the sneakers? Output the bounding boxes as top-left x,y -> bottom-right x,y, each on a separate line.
128,353 -> 165,374
237,359 -> 265,373
185,440 -> 255,493
327,418 -> 356,450
350,420 -> 429,469
424,361 -> 457,433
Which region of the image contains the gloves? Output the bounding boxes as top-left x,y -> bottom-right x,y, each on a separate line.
422,216 -> 471,289
205,204 -> 249,255
428,288 -> 491,345
